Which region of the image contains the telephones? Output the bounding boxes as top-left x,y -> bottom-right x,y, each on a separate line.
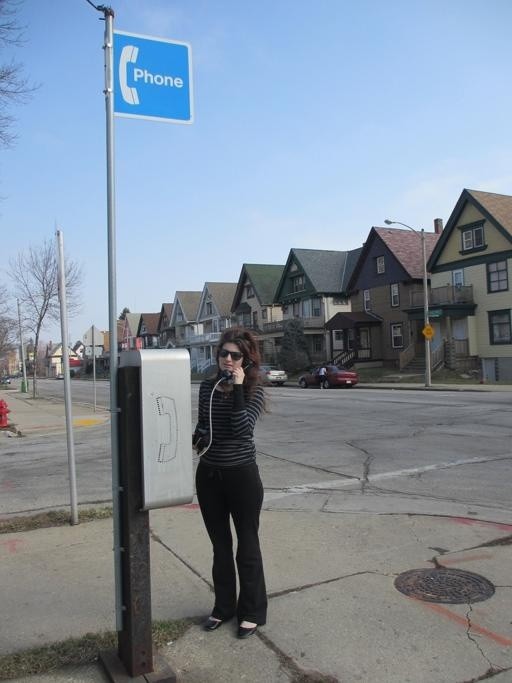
223,359 -> 254,379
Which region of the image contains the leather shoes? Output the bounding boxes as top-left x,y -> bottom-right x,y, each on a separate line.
237,621 -> 258,639
204,615 -> 225,631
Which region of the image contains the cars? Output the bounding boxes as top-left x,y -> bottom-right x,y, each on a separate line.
0,376 -> 11,384
298,365 -> 359,389
258,365 -> 288,386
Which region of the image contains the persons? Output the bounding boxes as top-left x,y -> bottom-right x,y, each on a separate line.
192,326 -> 271,637
319,366 -> 327,389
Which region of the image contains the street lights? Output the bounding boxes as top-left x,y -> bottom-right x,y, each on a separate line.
384,219 -> 432,386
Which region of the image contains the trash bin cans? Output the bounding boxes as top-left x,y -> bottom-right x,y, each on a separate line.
21,380 -> 28,392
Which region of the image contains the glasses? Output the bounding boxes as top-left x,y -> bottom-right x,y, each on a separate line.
218,348 -> 246,362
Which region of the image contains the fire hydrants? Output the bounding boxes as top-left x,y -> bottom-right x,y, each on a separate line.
0,399 -> 10,428
